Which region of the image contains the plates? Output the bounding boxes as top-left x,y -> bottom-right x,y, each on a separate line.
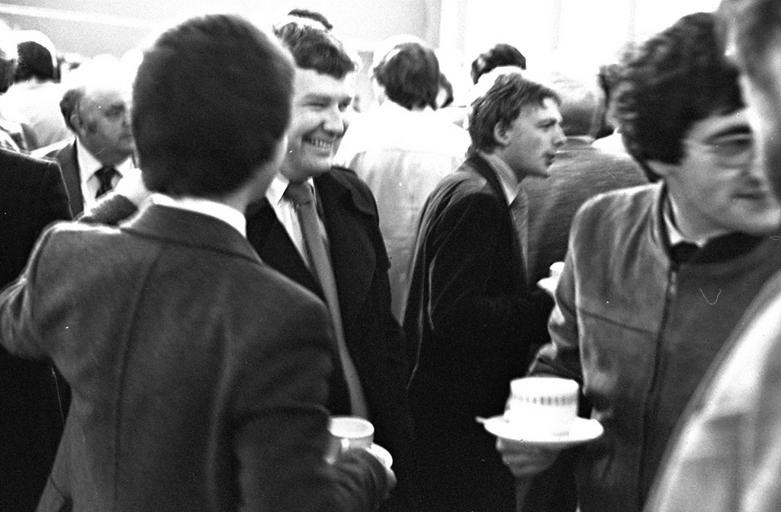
482,414 -> 606,449
326,441 -> 395,470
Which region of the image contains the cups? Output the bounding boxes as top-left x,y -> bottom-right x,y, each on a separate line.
325,415 -> 375,460
508,376 -> 580,435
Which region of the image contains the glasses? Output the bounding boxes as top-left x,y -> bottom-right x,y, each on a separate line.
678,135 -> 754,172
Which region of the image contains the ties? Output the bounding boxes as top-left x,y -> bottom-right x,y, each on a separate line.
96,166 -> 117,201
512,187 -> 529,285
285,181 -> 371,422
